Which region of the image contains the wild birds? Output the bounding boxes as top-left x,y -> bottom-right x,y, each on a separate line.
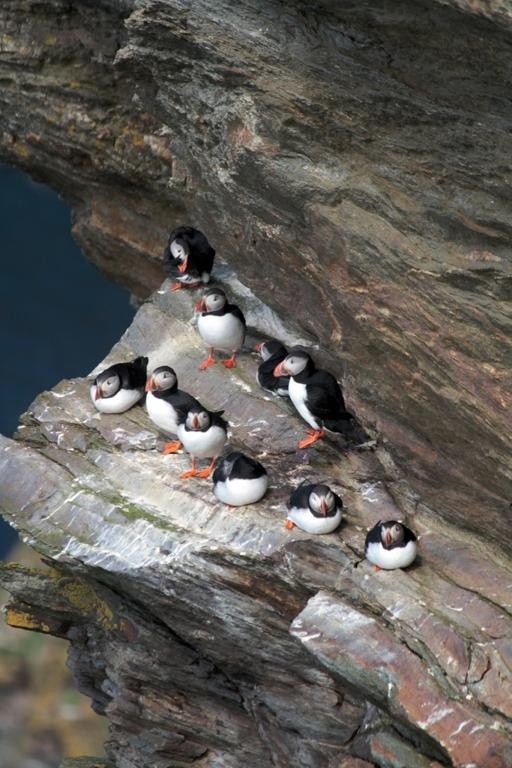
89,356 -> 148,414
145,366 -> 225,455
285,481 -> 343,535
212,451 -> 268,507
194,288 -> 247,370
162,226 -> 216,291
273,350 -> 355,449
364,520 -> 417,572
255,340 -> 292,397
177,407 -> 229,480
